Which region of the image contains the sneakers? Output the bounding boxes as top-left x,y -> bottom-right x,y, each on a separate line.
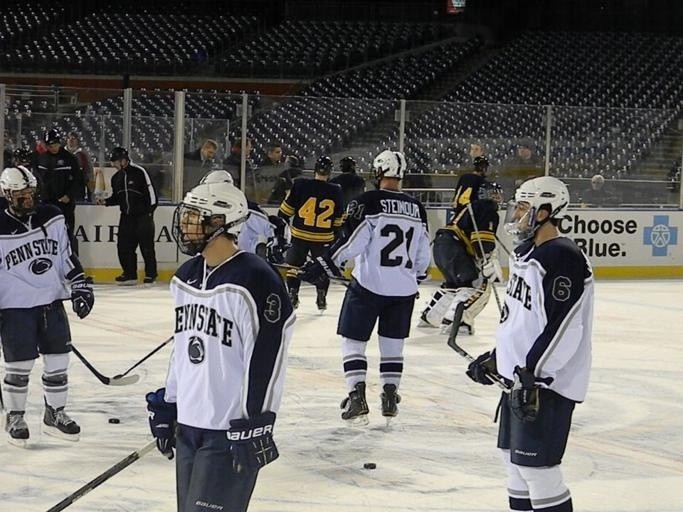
7,410 -> 30,439
317,295 -> 327,309
43,408 -> 80,434
115,272 -> 137,282
143,275 -> 159,283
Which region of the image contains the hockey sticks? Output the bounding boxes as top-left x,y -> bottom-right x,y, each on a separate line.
256,243 -> 350,283
448,302 -> 512,395
113,336 -> 172,379
71,345 -> 141,386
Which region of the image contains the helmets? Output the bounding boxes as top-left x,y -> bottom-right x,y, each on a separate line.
473,157 -> 504,205
503,176 -> 570,245
109,147 -> 128,162
0,166 -> 41,216
315,155 -> 356,175
44,129 -> 61,145
14,146 -> 34,163
369,150 -> 407,189
172,170 -> 251,256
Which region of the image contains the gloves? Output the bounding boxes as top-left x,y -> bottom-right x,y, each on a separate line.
146,389 -> 177,461
67,268 -> 94,318
481,253 -> 502,284
226,410 -> 279,476
511,365 -> 541,422
465,348 -> 501,386
297,254 -> 343,287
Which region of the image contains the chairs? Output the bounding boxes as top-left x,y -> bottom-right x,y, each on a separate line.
0,0 -> 683,207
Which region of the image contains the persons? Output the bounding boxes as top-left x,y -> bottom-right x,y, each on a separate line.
295,147 -> 430,420
183,140 -> 218,198
141,153 -> 166,198
465,176 -> 594,510
63,132 -> 91,199
37,129 -> 81,253
21,138 -> 48,174
419,182 -> 504,333
1,165 -> 94,440
278,155 -> 363,310
97,147 -> 158,287
224,137 -> 302,206
144,178 -> 297,510
452,156 -> 490,215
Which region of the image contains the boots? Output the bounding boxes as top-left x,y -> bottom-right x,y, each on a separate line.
380,383 -> 401,416
341,381 -> 369,419
